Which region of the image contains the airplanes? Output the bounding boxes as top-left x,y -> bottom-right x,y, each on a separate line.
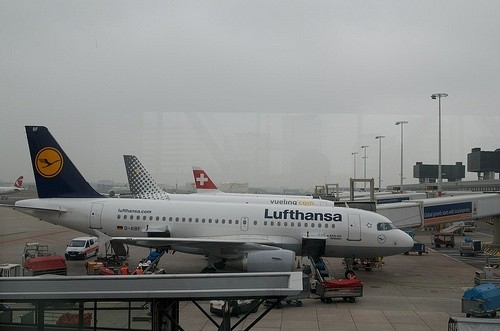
12,124 -> 417,285
0,176 -> 24,200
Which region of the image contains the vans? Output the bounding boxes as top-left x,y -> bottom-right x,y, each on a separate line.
64,236 -> 99,260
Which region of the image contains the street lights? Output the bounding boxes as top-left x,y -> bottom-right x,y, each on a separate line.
396,121 -> 408,189
376,135 -> 385,192
430,92 -> 448,192
361,145 -> 368,192
352,152 -> 358,190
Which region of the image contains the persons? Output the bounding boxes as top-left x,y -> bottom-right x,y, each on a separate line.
345,265 -> 356,279
133,245 -> 159,275
114,262 -> 131,276
